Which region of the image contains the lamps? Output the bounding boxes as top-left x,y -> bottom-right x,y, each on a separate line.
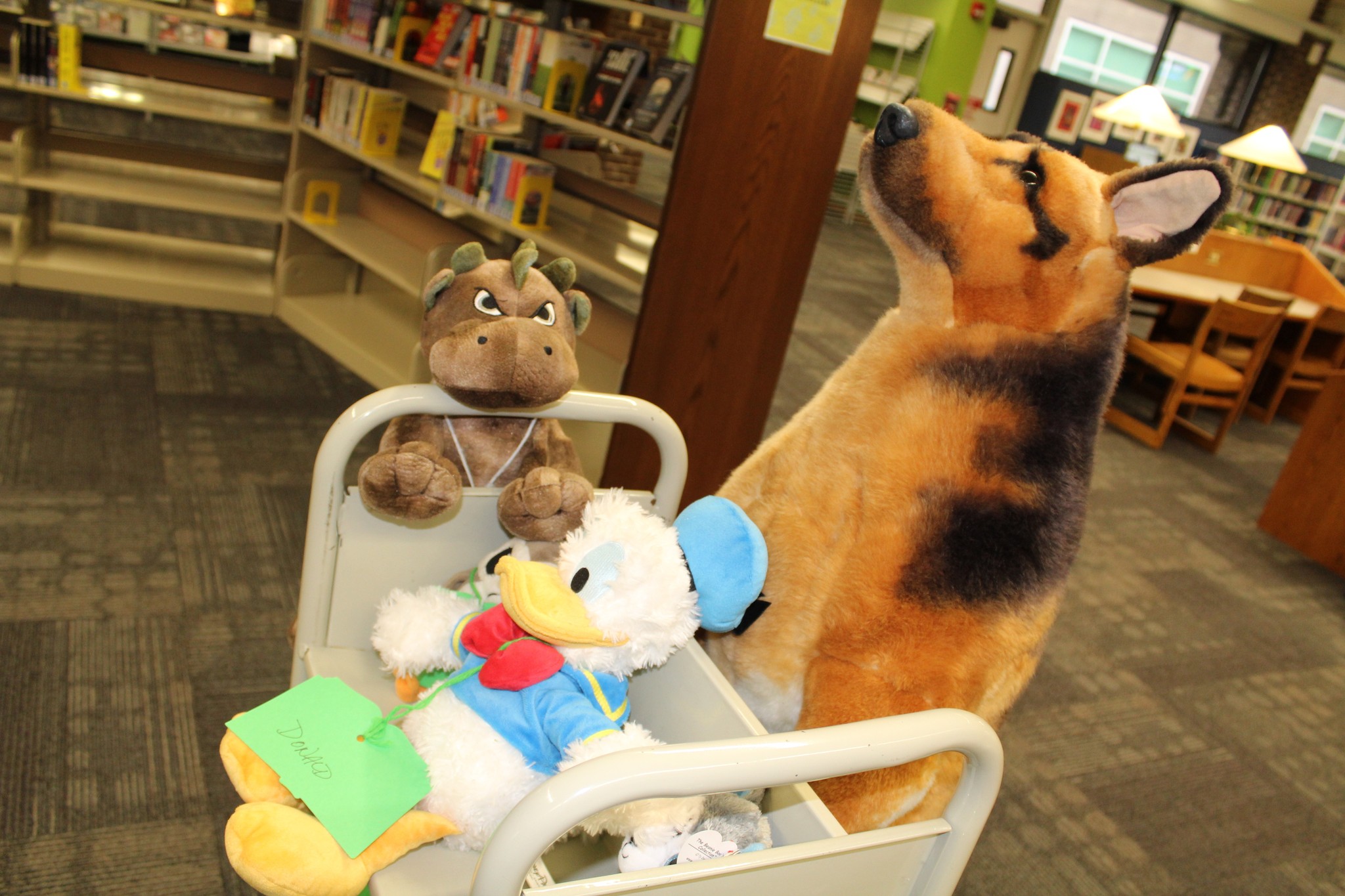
1218,124 -> 1309,175
1091,85 -> 1186,139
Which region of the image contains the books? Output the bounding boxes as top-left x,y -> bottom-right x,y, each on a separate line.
0,0 -> 698,234
1027,64 -> 1345,278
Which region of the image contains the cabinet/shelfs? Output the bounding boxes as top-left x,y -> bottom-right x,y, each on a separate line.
1199,141 -> 1345,281
276,0 -> 886,511
0,0 -> 302,319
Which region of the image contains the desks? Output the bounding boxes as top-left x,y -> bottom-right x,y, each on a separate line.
1132,267 -> 1326,441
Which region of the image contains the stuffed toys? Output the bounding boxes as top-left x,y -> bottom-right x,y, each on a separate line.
692,94 -> 1233,836
356,245 -> 596,538
217,478 -> 793,894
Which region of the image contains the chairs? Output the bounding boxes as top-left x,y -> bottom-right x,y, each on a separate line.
1106,285 -> 1345,447
